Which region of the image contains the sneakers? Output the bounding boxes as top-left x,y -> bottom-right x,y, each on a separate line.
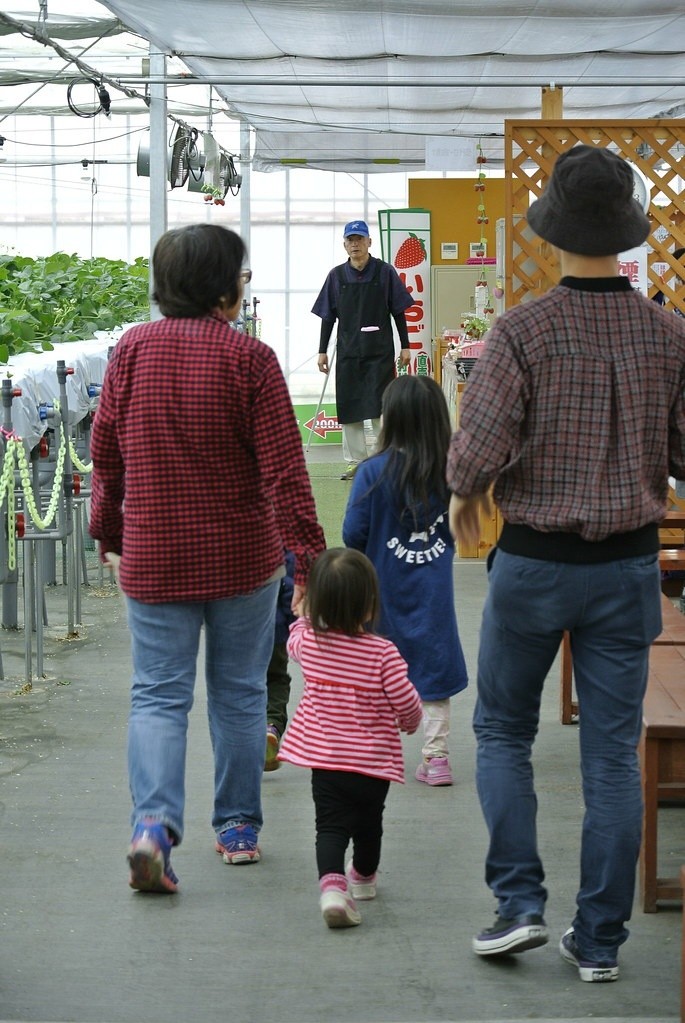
215,824 -> 261,863
559,927 -> 618,982
347,859 -> 377,900
320,873 -> 362,927
127,822 -> 176,893
415,758 -> 452,785
472,915 -> 548,955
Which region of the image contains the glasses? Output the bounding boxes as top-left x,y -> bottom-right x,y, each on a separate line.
222,268 -> 252,295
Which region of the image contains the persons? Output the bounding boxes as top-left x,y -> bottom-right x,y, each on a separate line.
286,548 -> 424,928
88,226 -> 326,893
444,144 -> 685,982
262,548 -> 298,771
311,219 -> 415,479
341,374 -> 469,786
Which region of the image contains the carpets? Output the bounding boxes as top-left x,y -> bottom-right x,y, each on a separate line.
306,463 -> 356,551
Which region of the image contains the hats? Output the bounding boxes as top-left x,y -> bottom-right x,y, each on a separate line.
344,221 -> 369,238
526,145 -> 651,255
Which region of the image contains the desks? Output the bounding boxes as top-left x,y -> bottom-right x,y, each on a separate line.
561,483 -> 684,913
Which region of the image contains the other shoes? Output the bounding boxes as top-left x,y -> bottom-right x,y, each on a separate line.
340,463 -> 358,481
263,726 -> 280,771
